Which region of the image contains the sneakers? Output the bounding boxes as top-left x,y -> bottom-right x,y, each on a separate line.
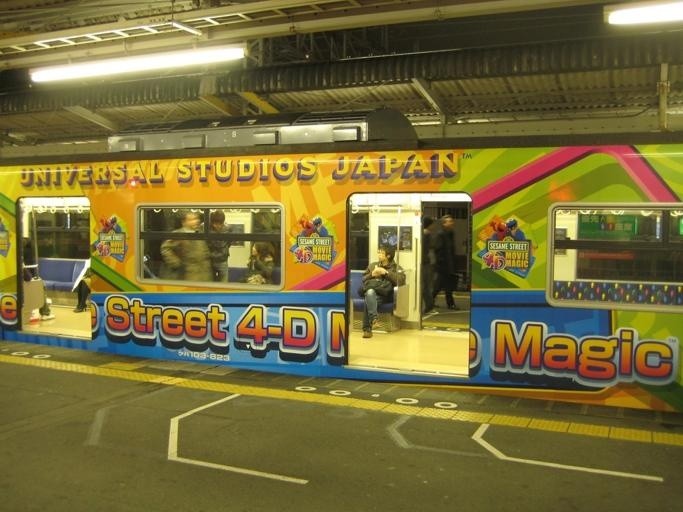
41,314 -> 55,320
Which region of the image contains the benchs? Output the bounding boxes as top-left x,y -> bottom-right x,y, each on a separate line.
38,257 -> 88,292
227,266 -> 397,333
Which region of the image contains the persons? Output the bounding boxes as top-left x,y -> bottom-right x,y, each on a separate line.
423,217 -> 439,316
202,211 -> 239,282
160,212 -> 213,281
237,241 -> 276,285
72,267 -> 90,313
358,246 -> 405,338
22,237 -> 56,323
432,214 -> 461,310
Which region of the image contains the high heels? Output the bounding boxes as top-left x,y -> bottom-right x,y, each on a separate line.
73,304 -> 87,312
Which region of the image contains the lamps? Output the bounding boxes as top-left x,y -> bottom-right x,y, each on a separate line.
28,39 -> 249,84
601,1 -> 683,24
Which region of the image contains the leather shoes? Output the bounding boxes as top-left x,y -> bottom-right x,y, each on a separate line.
371,320 -> 382,330
364,329 -> 372,339
422,300 -> 459,313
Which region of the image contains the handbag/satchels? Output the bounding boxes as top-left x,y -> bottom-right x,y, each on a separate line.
244,270 -> 267,285
358,278 -> 393,297
